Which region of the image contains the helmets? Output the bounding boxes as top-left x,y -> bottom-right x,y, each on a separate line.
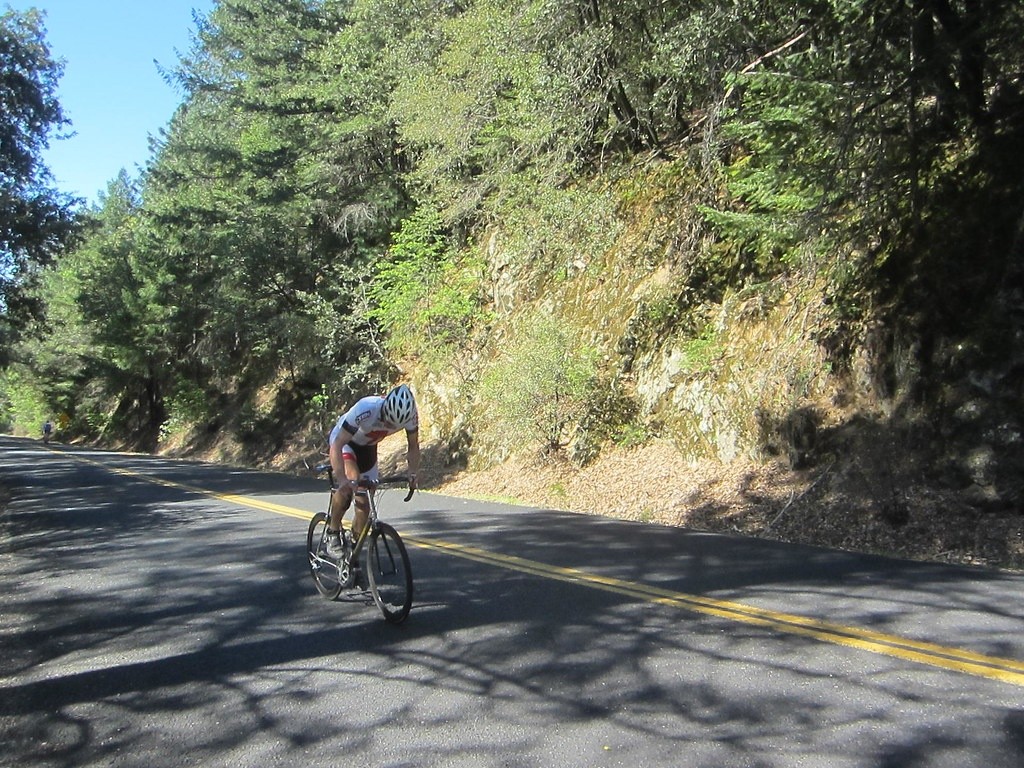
384,385 -> 414,423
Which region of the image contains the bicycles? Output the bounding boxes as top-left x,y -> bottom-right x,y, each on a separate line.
306,465 -> 416,626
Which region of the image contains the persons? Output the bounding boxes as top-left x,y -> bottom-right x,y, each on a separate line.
323,385 -> 422,557
44,420 -> 51,443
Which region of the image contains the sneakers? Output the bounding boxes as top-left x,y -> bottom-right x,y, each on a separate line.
356,568 -> 368,591
324,530 -> 345,561
